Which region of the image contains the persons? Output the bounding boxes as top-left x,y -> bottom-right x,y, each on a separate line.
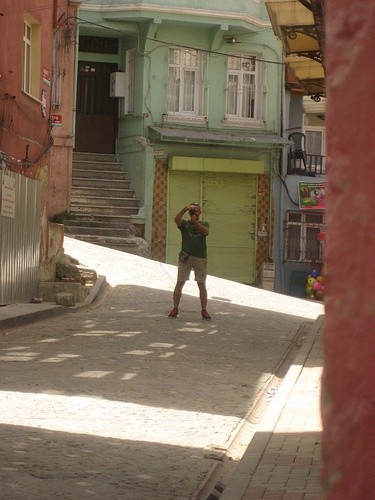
168,202 -> 212,320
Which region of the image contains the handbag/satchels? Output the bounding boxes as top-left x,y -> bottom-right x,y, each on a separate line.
179,250 -> 190,262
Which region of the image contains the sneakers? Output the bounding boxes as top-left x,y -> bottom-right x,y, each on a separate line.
169,308 -> 179,319
201,310 -> 211,320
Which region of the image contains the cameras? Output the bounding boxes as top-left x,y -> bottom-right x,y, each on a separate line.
189,210 -> 195,216
